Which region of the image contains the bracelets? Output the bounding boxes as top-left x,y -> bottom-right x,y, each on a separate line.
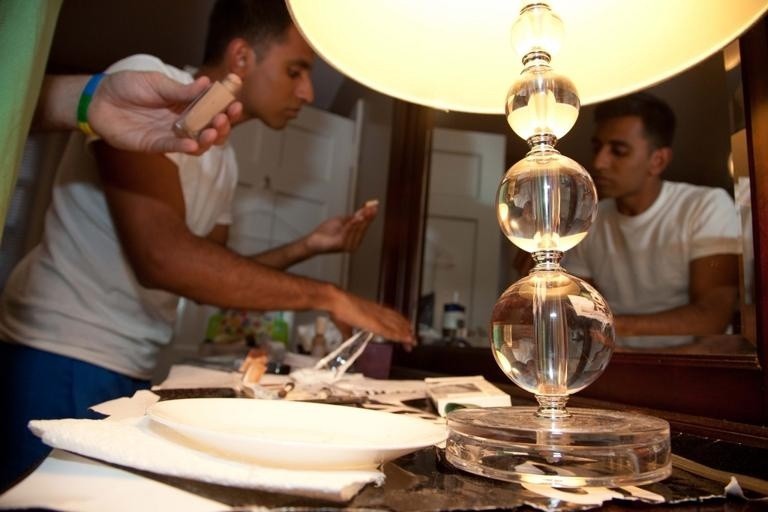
77,73 -> 107,145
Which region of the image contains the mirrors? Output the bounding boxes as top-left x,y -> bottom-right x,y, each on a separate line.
382,38 -> 764,372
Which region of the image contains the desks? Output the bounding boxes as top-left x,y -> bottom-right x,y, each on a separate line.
1,358 -> 768,512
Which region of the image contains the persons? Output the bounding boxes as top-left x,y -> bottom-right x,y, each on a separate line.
1,0 -> 416,488
512,92 -> 743,349
1,1 -> 243,239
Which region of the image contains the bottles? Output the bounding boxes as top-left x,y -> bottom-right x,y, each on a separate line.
171,72 -> 243,139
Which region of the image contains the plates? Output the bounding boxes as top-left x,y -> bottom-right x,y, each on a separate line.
145,397 -> 449,468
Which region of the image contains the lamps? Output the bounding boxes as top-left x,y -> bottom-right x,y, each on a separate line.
284,0 -> 768,489
